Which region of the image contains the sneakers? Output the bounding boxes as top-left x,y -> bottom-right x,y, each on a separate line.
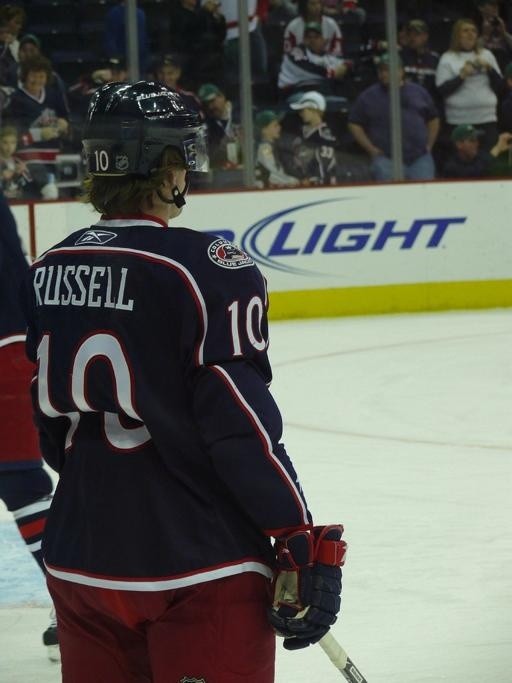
41,598 -> 67,649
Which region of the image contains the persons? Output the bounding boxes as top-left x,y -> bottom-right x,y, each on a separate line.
19,75 -> 352,682
0,1 -> 512,202
0,192 -> 60,662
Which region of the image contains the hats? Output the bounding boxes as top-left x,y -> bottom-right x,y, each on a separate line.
198,80 -> 219,104
291,89 -> 329,115
251,108 -> 275,129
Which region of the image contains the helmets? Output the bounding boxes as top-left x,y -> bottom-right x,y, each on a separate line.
80,75 -> 216,180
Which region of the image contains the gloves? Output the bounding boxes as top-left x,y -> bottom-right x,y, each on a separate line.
273,523 -> 351,647
